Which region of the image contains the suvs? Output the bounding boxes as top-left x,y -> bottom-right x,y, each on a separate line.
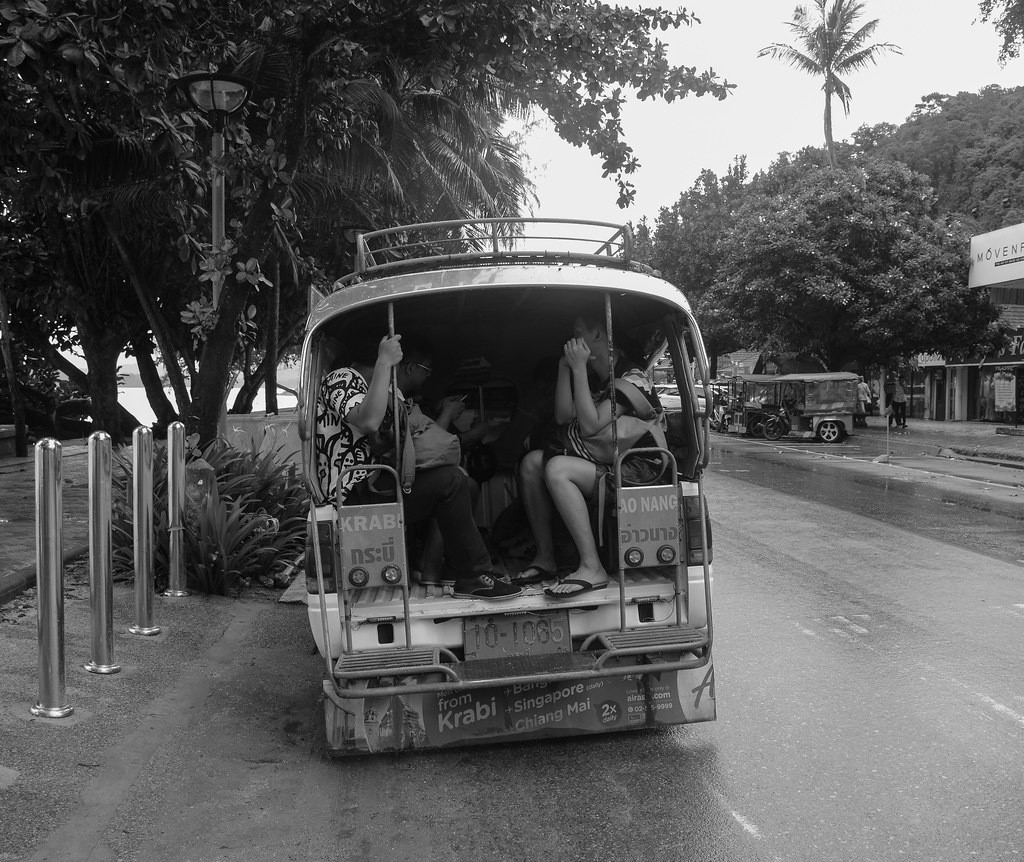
653,384 -> 734,415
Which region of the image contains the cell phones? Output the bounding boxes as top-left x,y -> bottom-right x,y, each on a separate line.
455,394 -> 467,402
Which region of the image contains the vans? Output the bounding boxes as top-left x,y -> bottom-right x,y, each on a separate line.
300,217 -> 718,761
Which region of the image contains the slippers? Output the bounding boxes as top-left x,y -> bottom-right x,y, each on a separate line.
548,569 -> 608,598
511,565 -> 573,584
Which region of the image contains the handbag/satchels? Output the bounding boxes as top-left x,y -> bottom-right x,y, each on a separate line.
365,389 -> 463,496
555,372 -> 670,489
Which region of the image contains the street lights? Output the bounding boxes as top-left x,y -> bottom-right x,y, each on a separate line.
184,32 -> 252,449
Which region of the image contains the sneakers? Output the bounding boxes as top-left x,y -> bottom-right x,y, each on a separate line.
421,571 -> 458,588
453,575 -> 521,599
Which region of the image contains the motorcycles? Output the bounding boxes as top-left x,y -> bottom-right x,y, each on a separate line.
715,373 -> 863,442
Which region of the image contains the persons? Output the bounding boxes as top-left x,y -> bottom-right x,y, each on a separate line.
857,375 -> 872,427
316,330 -> 523,601
883,371 -> 908,429
488,311 -> 667,599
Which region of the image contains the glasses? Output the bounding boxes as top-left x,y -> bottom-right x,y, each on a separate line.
401,360 -> 433,378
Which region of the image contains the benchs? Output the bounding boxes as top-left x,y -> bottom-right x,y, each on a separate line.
586,490 -> 621,575
